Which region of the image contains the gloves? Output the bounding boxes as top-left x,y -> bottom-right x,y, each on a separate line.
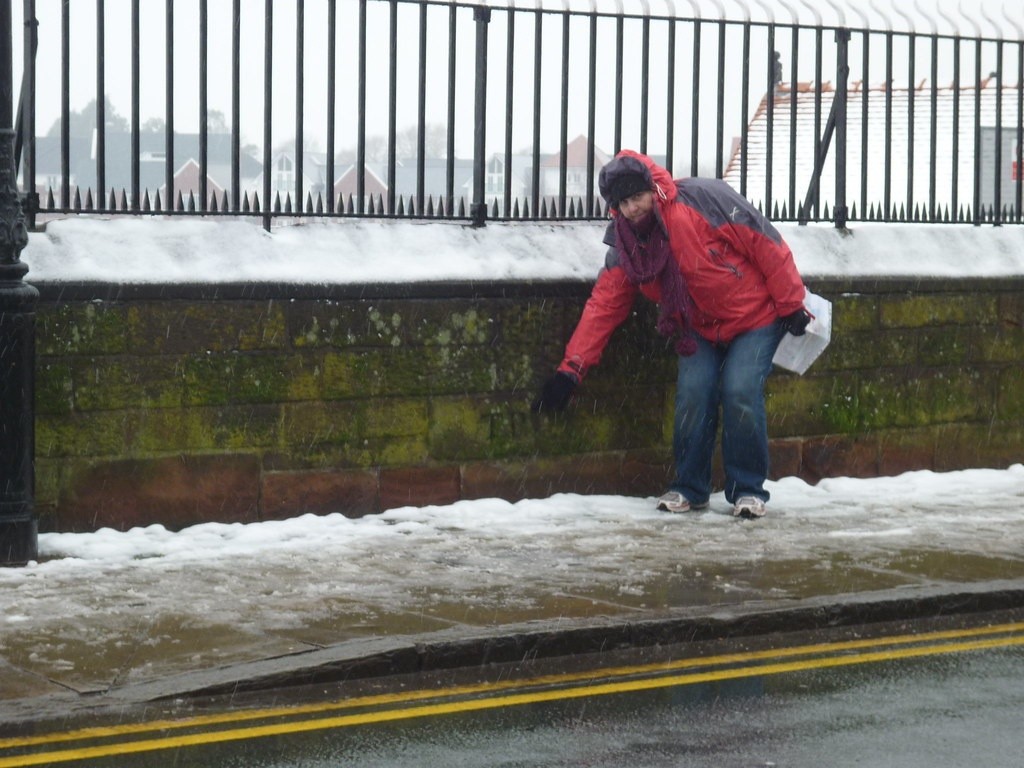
531,371 -> 574,412
782,310 -> 810,336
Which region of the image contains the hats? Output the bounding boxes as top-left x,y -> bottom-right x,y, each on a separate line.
613,174 -> 650,209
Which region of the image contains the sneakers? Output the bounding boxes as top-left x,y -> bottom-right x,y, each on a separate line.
656,491 -> 710,513
734,496 -> 766,518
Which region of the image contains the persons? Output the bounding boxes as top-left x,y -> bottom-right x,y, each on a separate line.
530,146 -> 817,518
